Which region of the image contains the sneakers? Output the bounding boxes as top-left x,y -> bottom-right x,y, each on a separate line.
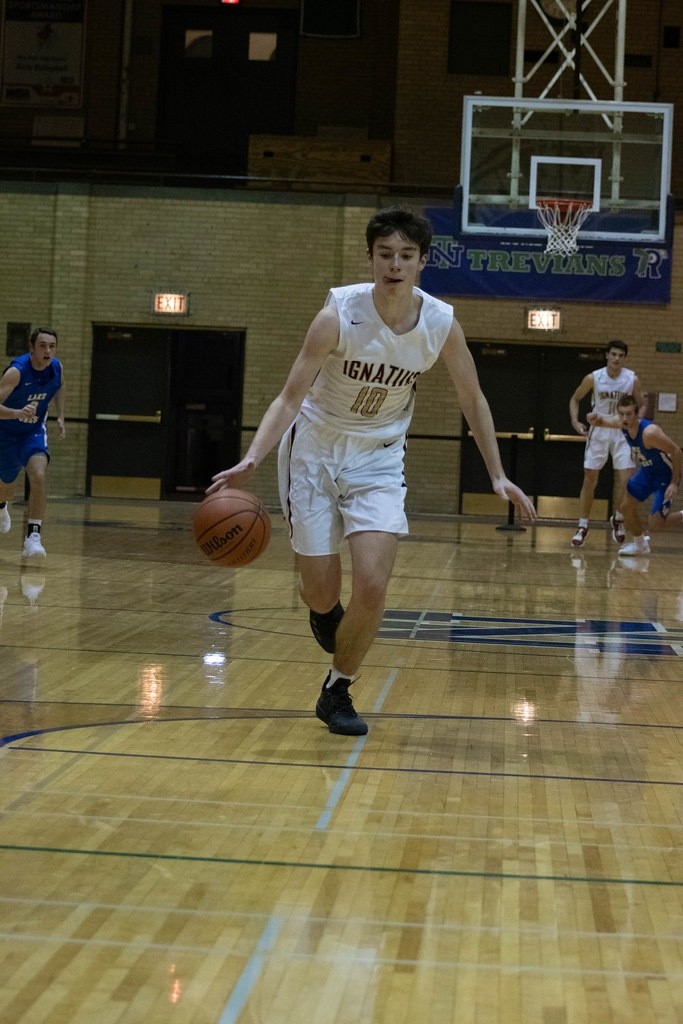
22,533 -> 47,559
0,501 -> 11,533
610,513 -> 626,544
309,600 -> 345,654
618,543 -> 651,555
315,669 -> 368,736
570,525 -> 589,548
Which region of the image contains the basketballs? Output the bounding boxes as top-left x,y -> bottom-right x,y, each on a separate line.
192,489 -> 271,566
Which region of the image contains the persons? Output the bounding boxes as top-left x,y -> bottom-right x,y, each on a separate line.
0,327 -> 65,560
205,205 -> 538,736
569,340 -> 683,557
